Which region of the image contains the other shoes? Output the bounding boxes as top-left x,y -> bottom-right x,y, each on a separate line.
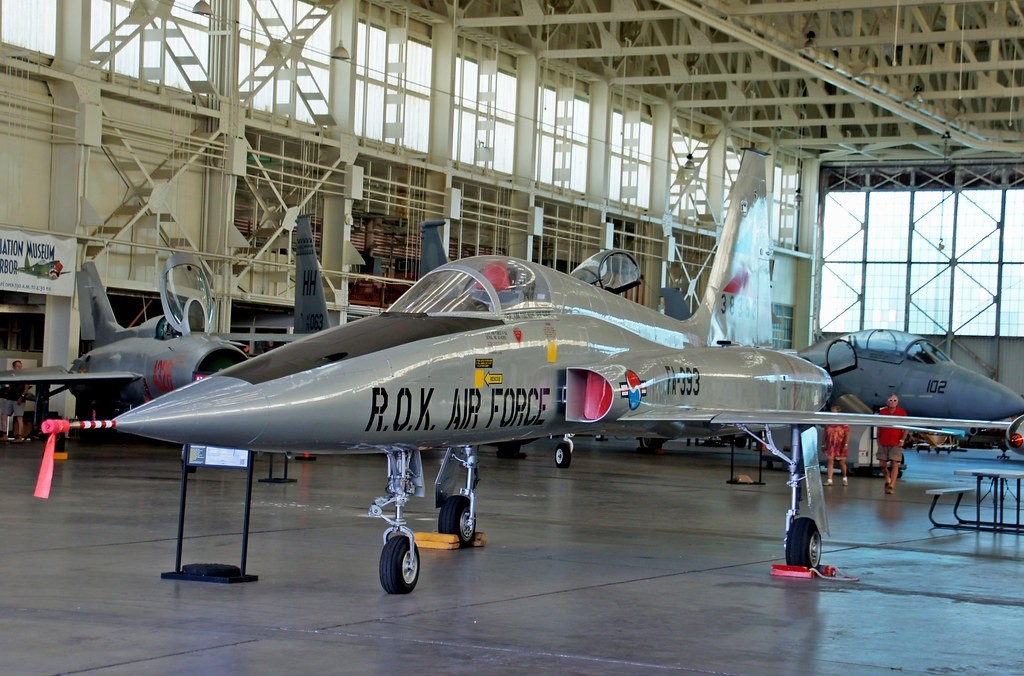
823,481 -> 833,486
12,436 -> 23,443
885,477 -> 891,485
886,486 -> 895,493
843,480 -> 848,485
0,434 -> 8,441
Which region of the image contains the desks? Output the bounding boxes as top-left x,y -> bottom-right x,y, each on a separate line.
953,467 -> 1024,533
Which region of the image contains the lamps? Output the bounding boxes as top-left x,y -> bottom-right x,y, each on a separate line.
191,0 -> 1024,201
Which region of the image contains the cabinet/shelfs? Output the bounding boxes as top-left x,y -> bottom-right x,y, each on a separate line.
924,487 -> 976,525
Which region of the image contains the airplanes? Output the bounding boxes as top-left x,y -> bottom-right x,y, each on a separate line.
0,146 -> 1024,598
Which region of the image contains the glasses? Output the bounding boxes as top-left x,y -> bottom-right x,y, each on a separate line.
888,399 -> 898,402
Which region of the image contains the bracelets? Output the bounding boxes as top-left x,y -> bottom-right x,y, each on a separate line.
22,394 -> 25,398
822,445 -> 824,447
900,440 -> 905,442
845,443 -> 849,446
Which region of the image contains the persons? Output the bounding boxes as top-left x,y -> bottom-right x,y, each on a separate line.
821,406 -> 850,486
876,394 -> 907,494
1,360 -> 29,443
